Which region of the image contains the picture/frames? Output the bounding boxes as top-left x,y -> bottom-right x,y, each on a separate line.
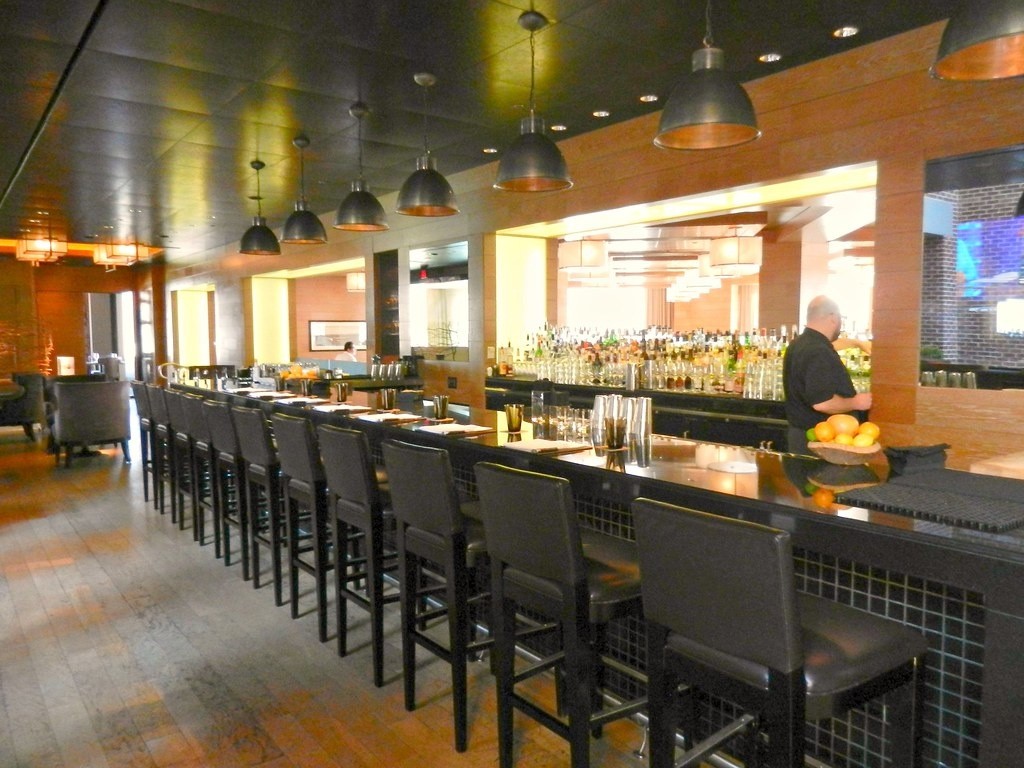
307,319 -> 368,352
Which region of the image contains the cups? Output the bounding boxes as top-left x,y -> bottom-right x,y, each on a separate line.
433,395 -> 449,419
530,391 -> 652,448
337,383 -> 348,402
963,371 -> 977,389
193,360 -> 342,391
935,370 -> 948,386
371,355 -> 418,382
921,371 -> 934,386
504,404 -> 524,433
380,388 -> 395,410
949,372 -> 961,387
508,433 -> 521,442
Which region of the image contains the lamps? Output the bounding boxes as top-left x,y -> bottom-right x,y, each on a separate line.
394,70 -> 461,218
87,242 -> 150,272
332,102 -> 392,233
653,0 -> 763,153
278,134 -> 328,246
237,158 -> 282,257
929,0 -> 1024,85
14,237 -> 69,267
664,227 -> 768,304
346,272 -> 365,294
493,12 -> 574,194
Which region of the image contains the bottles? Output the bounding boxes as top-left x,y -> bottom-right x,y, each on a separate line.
488,319 -> 873,402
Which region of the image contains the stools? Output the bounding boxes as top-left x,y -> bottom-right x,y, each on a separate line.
271,411 -> 429,645
627,492 -> 932,768
182,392 -> 266,557
162,387 -> 210,540
145,382 -> 173,524
131,378 -> 159,510
474,459 -> 697,768
382,432 -> 572,751
232,403 -> 364,607
313,418 -> 493,688
202,399 -> 295,580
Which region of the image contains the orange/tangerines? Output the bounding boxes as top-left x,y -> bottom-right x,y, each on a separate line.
280,364 -> 319,379
807,414 -> 880,447
813,488 -> 834,508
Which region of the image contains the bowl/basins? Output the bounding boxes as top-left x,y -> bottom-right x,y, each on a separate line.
807,461 -> 882,490
807,441 -> 880,465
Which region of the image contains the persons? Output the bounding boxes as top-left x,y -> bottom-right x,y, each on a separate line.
782,297 -> 873,455
334,342 -> 356,363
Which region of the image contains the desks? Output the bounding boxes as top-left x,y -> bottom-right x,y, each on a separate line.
86,360 -> 125,374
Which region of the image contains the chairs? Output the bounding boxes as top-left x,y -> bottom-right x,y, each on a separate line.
90,357 -> 121,381
0,370 -> 45,444
46,378 -> 135,470
